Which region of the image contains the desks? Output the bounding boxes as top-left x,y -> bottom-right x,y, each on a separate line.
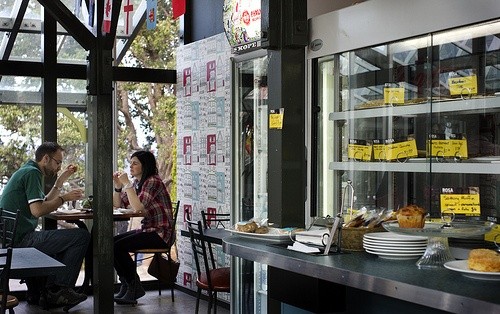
181,229 -> 228,244
0,247 -> 67,280
47,208 -> 145,292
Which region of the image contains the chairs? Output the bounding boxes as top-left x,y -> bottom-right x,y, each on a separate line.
0,202 -> 230,314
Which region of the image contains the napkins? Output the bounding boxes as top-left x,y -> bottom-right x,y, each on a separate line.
288,229 -> 331,254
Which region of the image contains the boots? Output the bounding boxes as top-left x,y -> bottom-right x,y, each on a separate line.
114,276 -> 146,304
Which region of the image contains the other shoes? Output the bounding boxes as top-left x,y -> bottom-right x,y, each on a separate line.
39,293 -> 55,310
46,287 -> 88,305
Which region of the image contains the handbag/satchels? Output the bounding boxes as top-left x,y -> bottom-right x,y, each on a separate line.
147,254 -> 180,284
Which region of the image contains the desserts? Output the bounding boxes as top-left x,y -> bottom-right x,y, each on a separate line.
396,204 -> 428,228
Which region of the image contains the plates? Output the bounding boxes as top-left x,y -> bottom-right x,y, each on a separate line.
362,232 -> 429,260
444,260 -> 500,281
223,222 -> 300,244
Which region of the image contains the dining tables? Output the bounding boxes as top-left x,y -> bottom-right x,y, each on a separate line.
222,234 -> 500,314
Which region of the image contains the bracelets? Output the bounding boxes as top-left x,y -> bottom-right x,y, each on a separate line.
124,181 -> 132,189
58,195 -> 65,205
115,188 -> 122,192
53,185 -> 62,191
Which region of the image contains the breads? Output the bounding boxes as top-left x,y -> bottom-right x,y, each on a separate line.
238,222 -> 269,234
469,249 -> 500,273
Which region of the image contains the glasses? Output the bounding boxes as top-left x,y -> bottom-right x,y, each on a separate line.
48,155 -> 62,166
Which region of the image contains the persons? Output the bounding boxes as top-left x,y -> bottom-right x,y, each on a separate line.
108,150 -> 176,304
0,142 -> 90,308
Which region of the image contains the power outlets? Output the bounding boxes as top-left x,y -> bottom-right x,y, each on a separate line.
184,273 -> 192,287
